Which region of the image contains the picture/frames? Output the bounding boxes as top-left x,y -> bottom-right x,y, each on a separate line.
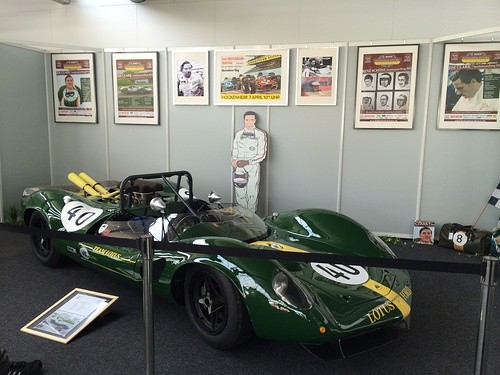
50,52 -> 98,124
111,52 -> 160,126
296,48 -> 339,106
172,51 -> 210,106
353,44 -> 420,131
435,40 -> 500,132
20,287 -> 119,345
213,49 -> 289,107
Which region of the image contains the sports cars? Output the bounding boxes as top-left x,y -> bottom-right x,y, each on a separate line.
22,171 -> 413,362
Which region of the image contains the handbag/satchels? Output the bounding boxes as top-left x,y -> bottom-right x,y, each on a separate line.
439,223 -> 492,255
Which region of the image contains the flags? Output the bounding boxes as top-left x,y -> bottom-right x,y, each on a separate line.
488,182 -> 500,209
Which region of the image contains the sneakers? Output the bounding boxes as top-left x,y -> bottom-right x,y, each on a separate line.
0,360 -> 42,375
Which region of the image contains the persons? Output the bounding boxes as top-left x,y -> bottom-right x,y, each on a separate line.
308,58 -> 316,68
179,62 -> 204,96
417,228 -> 434,245
450,68 -> 500,112
58,75 -> 83,108
